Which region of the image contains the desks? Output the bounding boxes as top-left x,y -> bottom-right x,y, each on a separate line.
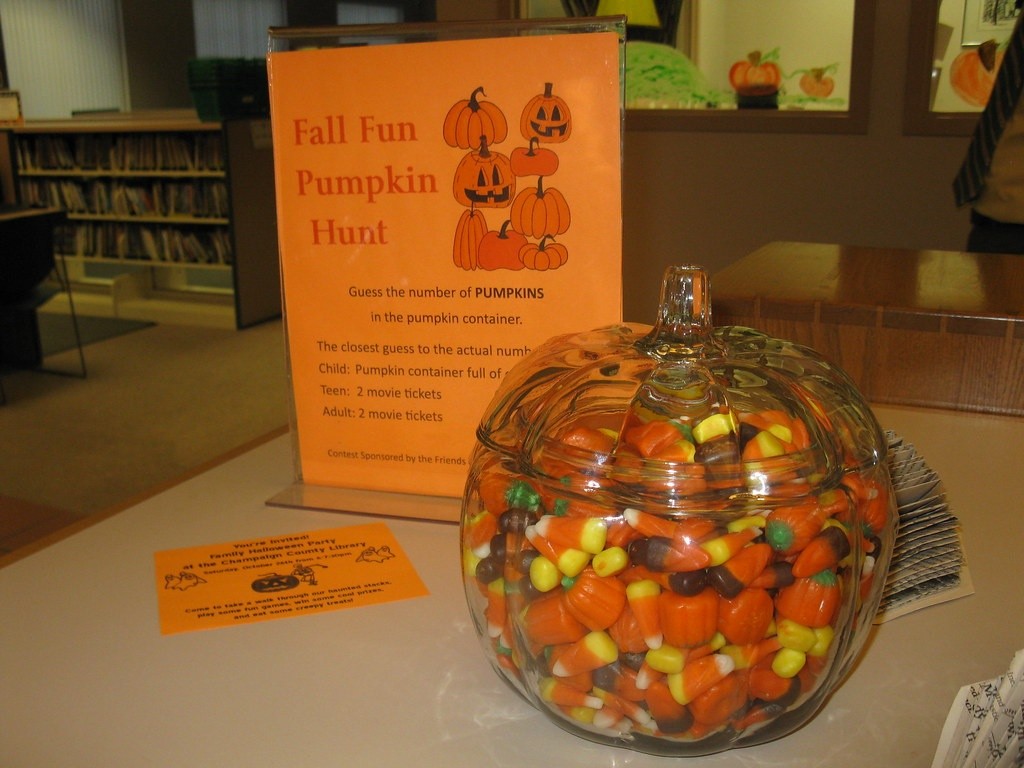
0,206 -> 88,405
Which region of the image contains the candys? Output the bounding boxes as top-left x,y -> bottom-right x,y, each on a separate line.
464,409 -> 890,738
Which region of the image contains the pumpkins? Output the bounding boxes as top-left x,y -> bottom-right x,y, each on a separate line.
443,86 -> 508,150
453,176 -> 571,271
510,137 -> 559,176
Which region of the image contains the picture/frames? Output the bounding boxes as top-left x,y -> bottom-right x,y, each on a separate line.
903,0 -> 1024,140
501,1 -> 877,135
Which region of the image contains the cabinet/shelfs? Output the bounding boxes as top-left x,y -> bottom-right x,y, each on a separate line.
710,239 -> 1024,419
0,109 -> 235,306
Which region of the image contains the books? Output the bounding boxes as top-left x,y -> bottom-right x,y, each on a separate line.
873,429 -> 975,625
13,136 -> 232,267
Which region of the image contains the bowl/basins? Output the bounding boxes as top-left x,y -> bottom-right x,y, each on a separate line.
459,264 -> 900,761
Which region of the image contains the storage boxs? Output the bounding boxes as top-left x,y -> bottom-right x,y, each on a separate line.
459,261 -> 898,761
